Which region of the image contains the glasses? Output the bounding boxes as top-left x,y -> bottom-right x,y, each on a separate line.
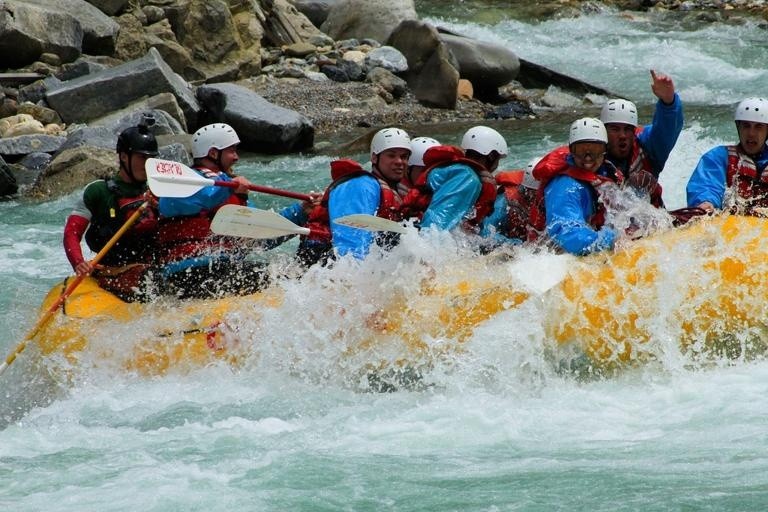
569,140 -> 608,160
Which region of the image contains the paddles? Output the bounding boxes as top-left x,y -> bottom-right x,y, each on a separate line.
146,159 -> 319,199
333,213 -> 408,235
209,204 -> 329,238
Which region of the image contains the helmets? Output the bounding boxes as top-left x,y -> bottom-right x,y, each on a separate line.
192,123 -> 241,158
601,99 -> 638,128
116,125 -> 159,156
735,97 -> 768,124
570,117 -> 608,145
370,127 -> 442,167
461,126 -> 508,158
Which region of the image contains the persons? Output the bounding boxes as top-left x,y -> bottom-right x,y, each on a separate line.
685,94 -> 768,216
329,64 -> 685,263
63,112 -> 323,299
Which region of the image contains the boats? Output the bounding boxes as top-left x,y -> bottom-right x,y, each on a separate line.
38,215 -> 764,384
